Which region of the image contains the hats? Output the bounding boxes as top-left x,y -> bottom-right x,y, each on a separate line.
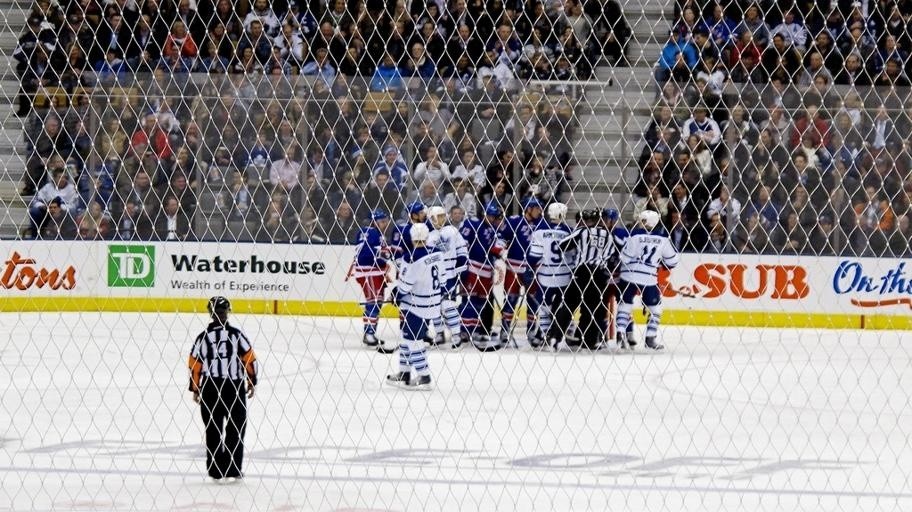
385,144 -> 398,156
351,145 -> 366,161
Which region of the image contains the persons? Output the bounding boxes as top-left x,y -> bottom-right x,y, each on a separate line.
356,204 -> 678,389
187,295 -> 258,483
10,2 -> 911,259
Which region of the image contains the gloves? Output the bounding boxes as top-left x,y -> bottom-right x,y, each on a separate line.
500,250 -> 508,260
245,385 -> 255,398
390,289 -> 402,306
193,391 -> 199,404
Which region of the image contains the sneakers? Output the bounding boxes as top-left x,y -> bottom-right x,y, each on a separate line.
362,333 -> 379,345
482,329 -> 498,337
626,333 -> 637,346
435,331 -> 445,346
226,473 -> 244,483
645,336 -> 664,349
572,336 -> 601,349
374,339 -> 384,344
462,333 -> 469,344
546,336 -> 558,351
215,476 -> 225,483
450,334 -> 462,351
616,330 -> 630,351
386,371 -> 410,386
528,334 -> 544,349
405,375 -> 431,390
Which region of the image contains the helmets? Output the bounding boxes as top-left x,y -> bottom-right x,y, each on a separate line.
207,297 -> 231,315
548,202 -> 568,219
520,196 -> 544,206
483,203 -> 500,216
604,208 -> 618,220
638,211 -> 659,229
576,210 -> 592,222
591,207 -> 607,219
406,200 -> 425,214
409,223 -> 429,240
369,208 -> 389,219
427,206 -> 445,217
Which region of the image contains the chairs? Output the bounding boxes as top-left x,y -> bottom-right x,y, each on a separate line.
200,191 -> 228,214
228,216 -> 256,241
207,165 -> 233,182
250,166 -> 267,186
200,214 -> 226,241
472,119 -> 499,142
